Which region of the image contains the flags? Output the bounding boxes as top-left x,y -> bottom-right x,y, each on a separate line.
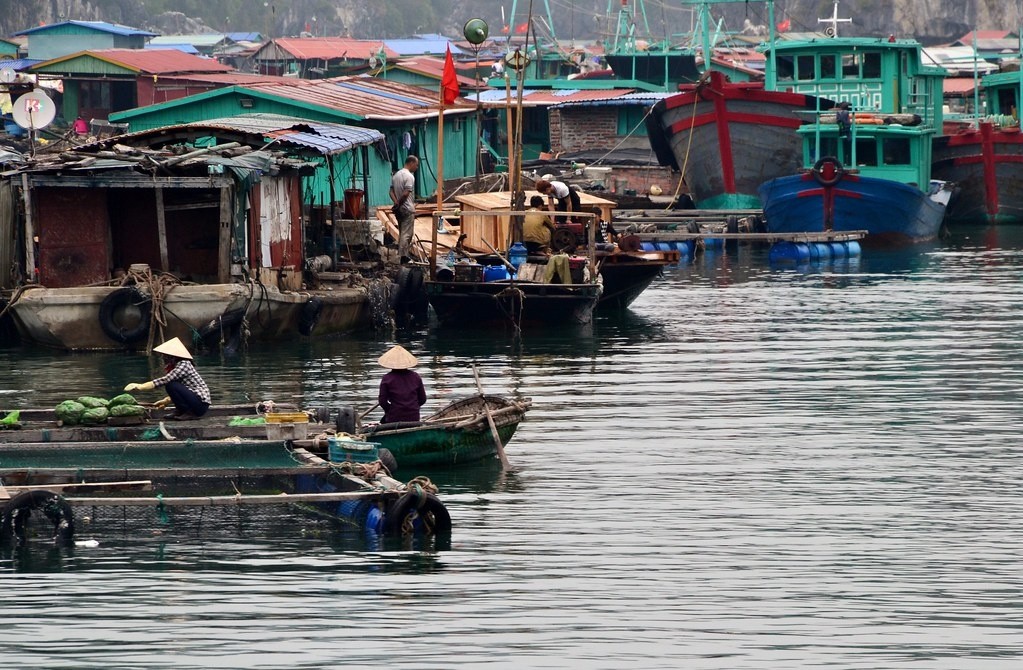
499,24 -> 510,35
439,41 -> 460,110
515,23 -> 528,34
777,19 -> 790,32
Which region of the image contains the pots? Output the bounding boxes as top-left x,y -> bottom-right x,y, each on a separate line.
569,255 -> 588,269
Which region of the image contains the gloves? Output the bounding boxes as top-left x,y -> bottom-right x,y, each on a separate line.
154,396 -> 171,409
123,381 -> 155,392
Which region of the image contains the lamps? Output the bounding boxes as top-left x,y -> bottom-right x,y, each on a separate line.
241,97 -> 255,108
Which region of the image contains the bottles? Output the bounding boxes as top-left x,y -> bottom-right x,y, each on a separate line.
438,216 -> 443,230
509,242 -> 527,280
34,268 -> 40,283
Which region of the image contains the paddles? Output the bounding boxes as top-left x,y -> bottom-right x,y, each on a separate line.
480,236 -> 518,272
139,402 -> 163,407
471,363 -> 516,471
359,402 -> 381,419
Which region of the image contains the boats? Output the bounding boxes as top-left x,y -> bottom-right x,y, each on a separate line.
10,112 -> 404,349
1,388 -> 534,479
420,0 -> 1023,341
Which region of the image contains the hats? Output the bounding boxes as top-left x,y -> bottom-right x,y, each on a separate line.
153,337 -> 193,359
378,345 -> 418,369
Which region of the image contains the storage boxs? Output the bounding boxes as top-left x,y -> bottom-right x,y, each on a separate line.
328,438 -> 382,464
454,264 -> 483,282
265,412 -> 309,442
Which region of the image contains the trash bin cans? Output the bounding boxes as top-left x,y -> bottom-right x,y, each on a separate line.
344,189 -> 364,220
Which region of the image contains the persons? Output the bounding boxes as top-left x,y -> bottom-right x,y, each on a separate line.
536,180 -> 580,224
584,207 -> 622,252
124,337 -> 212,421
491,60 -> 504,77
522,196 -> 558,256
378,344 -> 427,424
388,155 -> 418,264
73,115 -> 89,138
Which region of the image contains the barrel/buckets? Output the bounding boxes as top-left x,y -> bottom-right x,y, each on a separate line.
131,264 -> 149,282
344,189 -> 363,219
623,189 -> 630,196
434,265 -> 453,282
630,189 -> 636,197
484,265 -> 507,282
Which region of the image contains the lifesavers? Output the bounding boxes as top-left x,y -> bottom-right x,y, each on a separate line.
387,490 -> 454,537
747,215 -> 766,231
98,288 -> 152,343
193,308 -> 245,355
685,219 -> 707,252
813,156 -> 844,187
401,266 -> 424,299
727,214 -> 739,234
298,297 -> 324,336
0,488 -> 77,549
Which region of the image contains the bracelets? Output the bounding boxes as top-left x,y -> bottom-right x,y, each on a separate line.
567,218 -> 571,220
